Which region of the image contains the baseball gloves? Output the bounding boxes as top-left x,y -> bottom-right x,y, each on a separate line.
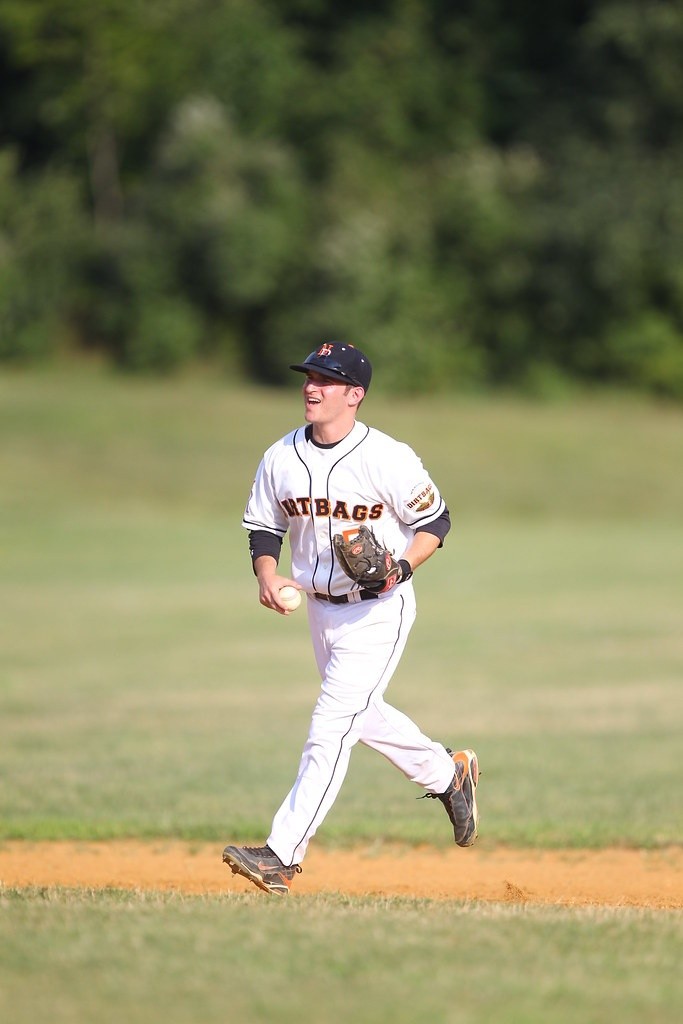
330,524 -> 403,597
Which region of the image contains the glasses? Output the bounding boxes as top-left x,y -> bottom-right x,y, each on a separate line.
304,351 -> 362,387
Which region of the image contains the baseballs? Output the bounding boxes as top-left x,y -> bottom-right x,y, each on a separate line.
278,586 -> 303,613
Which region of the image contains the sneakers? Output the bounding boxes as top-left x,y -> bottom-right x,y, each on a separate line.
416,748 -> 482,847
222,844 -> 303,896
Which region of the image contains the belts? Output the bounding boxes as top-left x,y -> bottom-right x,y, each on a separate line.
315,589 -> 378,604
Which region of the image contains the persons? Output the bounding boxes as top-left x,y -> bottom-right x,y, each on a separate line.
222,341 -> 478,896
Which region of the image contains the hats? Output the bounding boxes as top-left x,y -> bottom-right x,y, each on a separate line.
289,342 -> 372,391
